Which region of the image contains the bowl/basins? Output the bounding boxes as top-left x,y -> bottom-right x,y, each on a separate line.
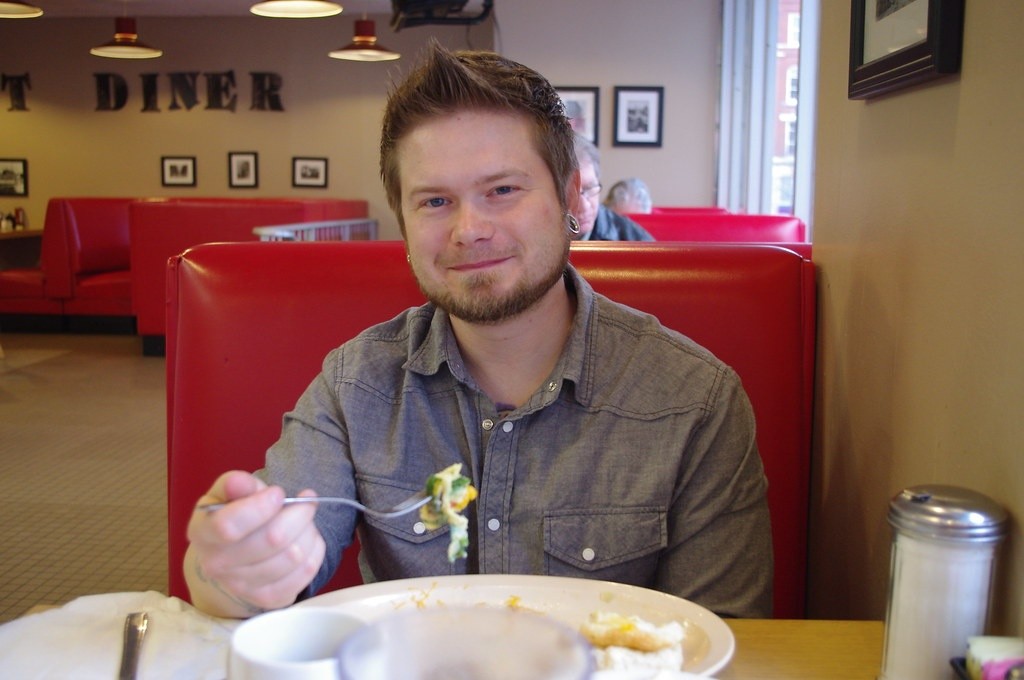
337,605 -> 596,680
266,575 -> 735,680
227,606 -> 386,680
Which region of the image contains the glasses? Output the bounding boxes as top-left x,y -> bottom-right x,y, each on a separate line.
578,180 -> 603,199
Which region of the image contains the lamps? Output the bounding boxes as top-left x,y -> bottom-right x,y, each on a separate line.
0,0 -> 44,18
250,0 -> 343,18
328,0 -> 400,60
90,0 -> 163,58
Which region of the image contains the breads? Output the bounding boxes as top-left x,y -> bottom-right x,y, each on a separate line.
577,609 -> 676,651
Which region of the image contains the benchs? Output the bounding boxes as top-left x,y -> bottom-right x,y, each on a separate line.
0,194 -> 813,619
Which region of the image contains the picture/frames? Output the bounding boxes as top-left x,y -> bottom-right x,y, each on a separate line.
292,157 -> 327,187
847,0 -> 967,100
228,151 -> 258,187
614,86 -> 663,145
0,158 -> 29,196
553,87 -> 599,145
160,156 -> 196,186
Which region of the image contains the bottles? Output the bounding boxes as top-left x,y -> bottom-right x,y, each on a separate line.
877,484 -> 1011,680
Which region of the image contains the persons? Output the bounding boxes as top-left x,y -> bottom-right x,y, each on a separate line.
572,130 -> 656,242
181,35 -> 777,620
607,177 -> 653,215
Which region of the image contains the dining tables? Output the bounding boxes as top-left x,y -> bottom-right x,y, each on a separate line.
717,618 -> 885,679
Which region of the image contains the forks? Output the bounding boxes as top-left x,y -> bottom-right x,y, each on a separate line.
196,487 -> 433,520
